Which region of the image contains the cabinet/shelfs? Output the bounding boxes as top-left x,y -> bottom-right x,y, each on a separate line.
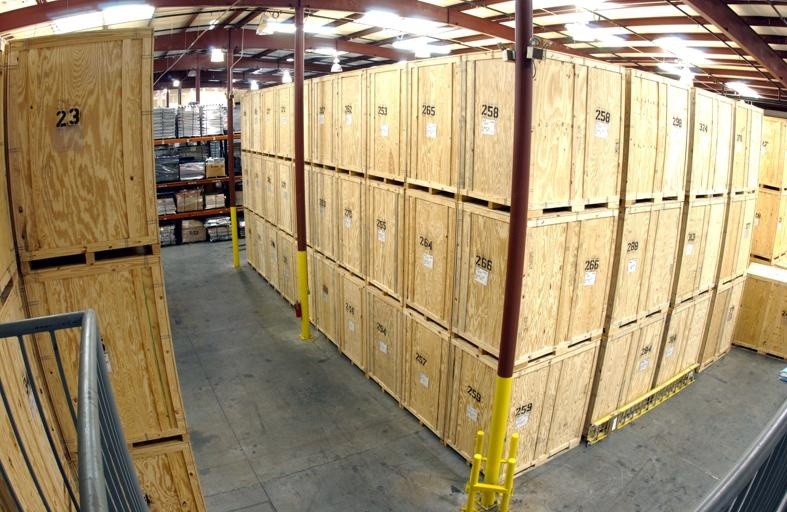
155,132 -> 243,221
